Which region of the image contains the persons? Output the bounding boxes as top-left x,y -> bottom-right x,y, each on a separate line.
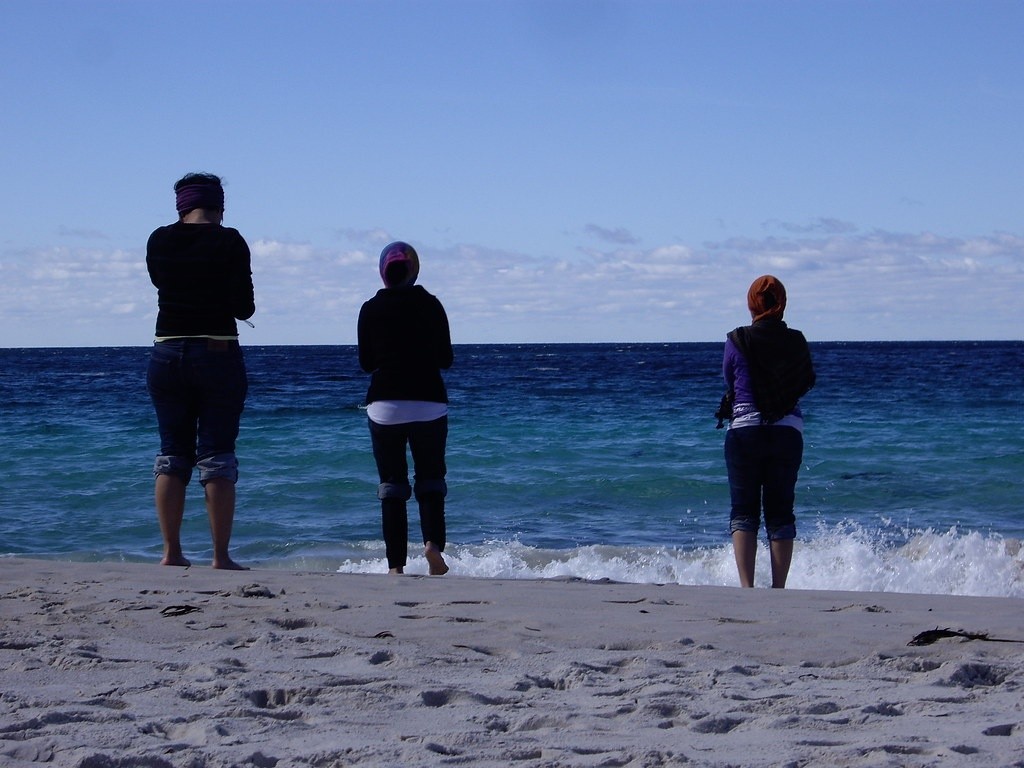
714,274 -> 816,589
357,240 -> 451,575
145,173 -> 256,570
